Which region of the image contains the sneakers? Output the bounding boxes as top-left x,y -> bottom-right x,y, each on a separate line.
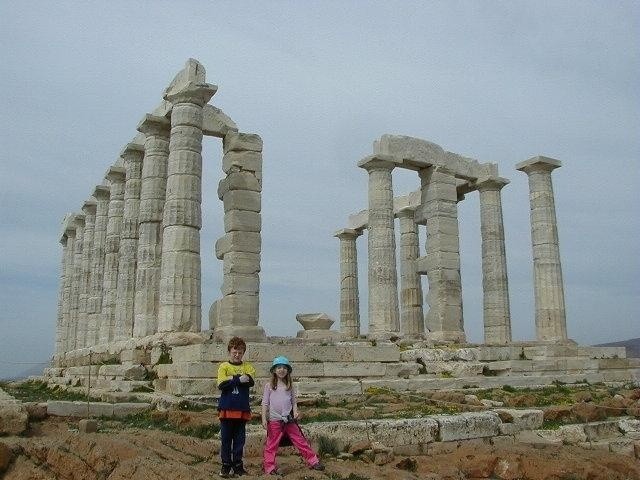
218,462 -> 232,477
270,469 -> 280,475
310,463 -> 325,471
232,462 -> 250,477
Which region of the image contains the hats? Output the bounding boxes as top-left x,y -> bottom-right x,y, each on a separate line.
269,356 -> 293,375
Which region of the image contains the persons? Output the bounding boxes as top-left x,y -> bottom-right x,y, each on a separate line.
261,356 -> 325,476
217,336 -> 257,477
